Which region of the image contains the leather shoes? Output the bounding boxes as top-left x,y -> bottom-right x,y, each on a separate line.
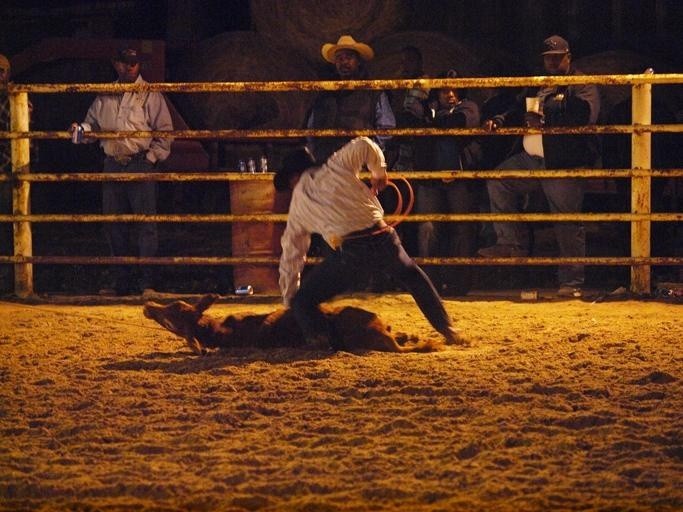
98,288 -> 116,296
446,333 -> 472,346
478,244 -> 531,258
557,286 -> 581,297
142,288 -> 158,297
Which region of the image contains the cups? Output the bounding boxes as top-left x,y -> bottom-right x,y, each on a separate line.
526,96 -> 540,112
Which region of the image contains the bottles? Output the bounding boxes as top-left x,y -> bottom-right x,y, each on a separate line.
239,155 -> 268,172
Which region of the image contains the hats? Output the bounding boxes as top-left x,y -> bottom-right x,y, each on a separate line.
573,36 -> 604,59
111,46 -> 145,63
539,34 -> 570,57
321,35 -> 374,64
273,147 -> 322,191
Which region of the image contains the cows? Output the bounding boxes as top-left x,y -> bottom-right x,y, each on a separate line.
143,294 -> 446,355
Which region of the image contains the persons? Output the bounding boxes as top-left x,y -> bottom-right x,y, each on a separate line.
264,137 -> 472,347
0,35 -> 601,294
